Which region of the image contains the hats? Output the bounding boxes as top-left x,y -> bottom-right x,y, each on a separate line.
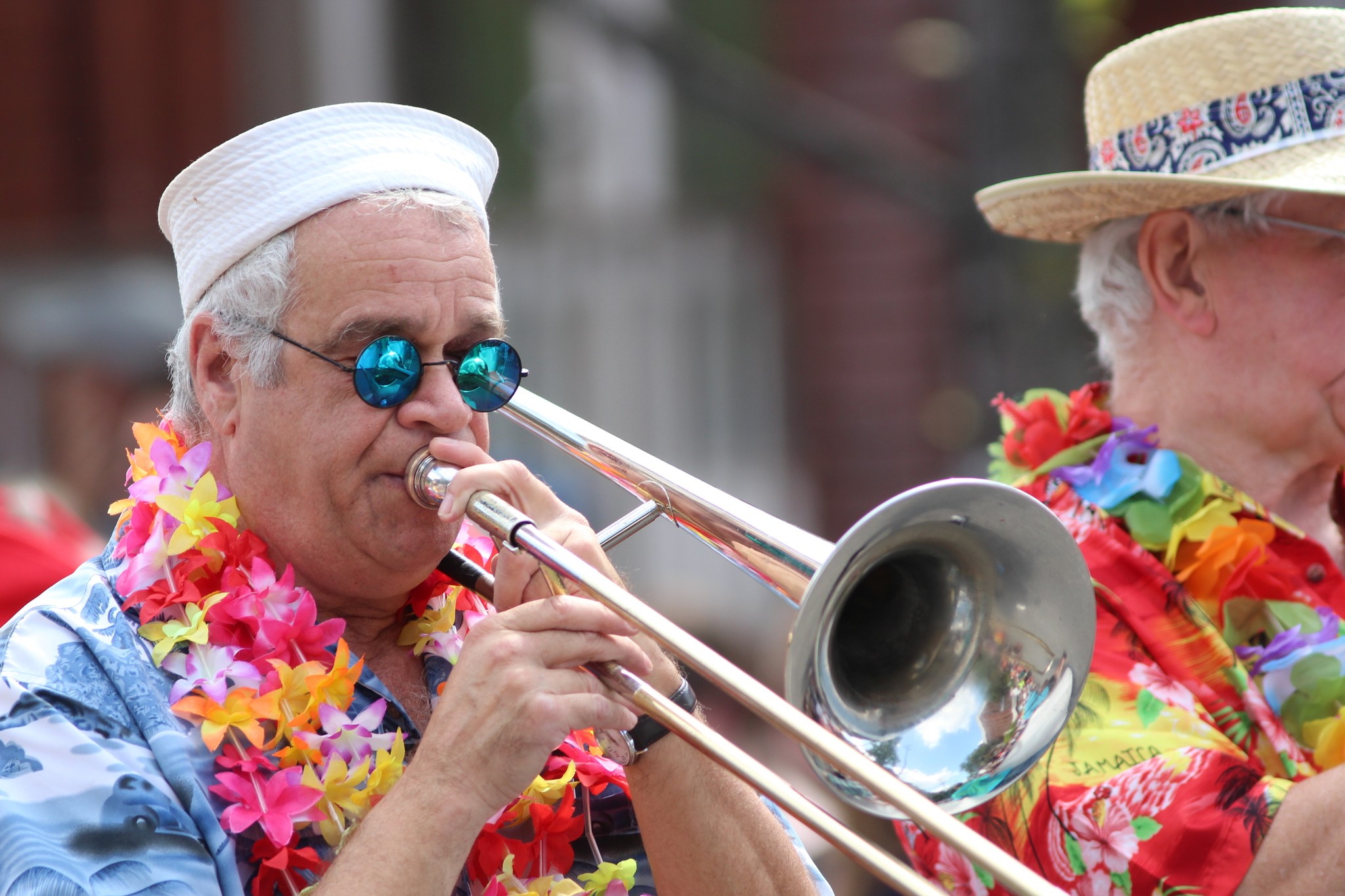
157,102 -> 499,322
972,7 -> 1344,241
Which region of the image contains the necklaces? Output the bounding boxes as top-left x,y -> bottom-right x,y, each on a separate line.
986,383 -> 1345,778
106,411 -> 638,896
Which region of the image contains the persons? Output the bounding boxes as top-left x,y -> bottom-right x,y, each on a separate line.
887,8 -> 1345,896
1,104 -> 832,896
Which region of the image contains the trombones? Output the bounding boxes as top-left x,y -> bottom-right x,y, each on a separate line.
405,352 -> 1097,895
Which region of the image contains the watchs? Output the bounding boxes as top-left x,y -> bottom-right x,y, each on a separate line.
593,673 -> 695,767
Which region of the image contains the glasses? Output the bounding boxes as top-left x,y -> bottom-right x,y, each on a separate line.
219,310 -> 528,413
